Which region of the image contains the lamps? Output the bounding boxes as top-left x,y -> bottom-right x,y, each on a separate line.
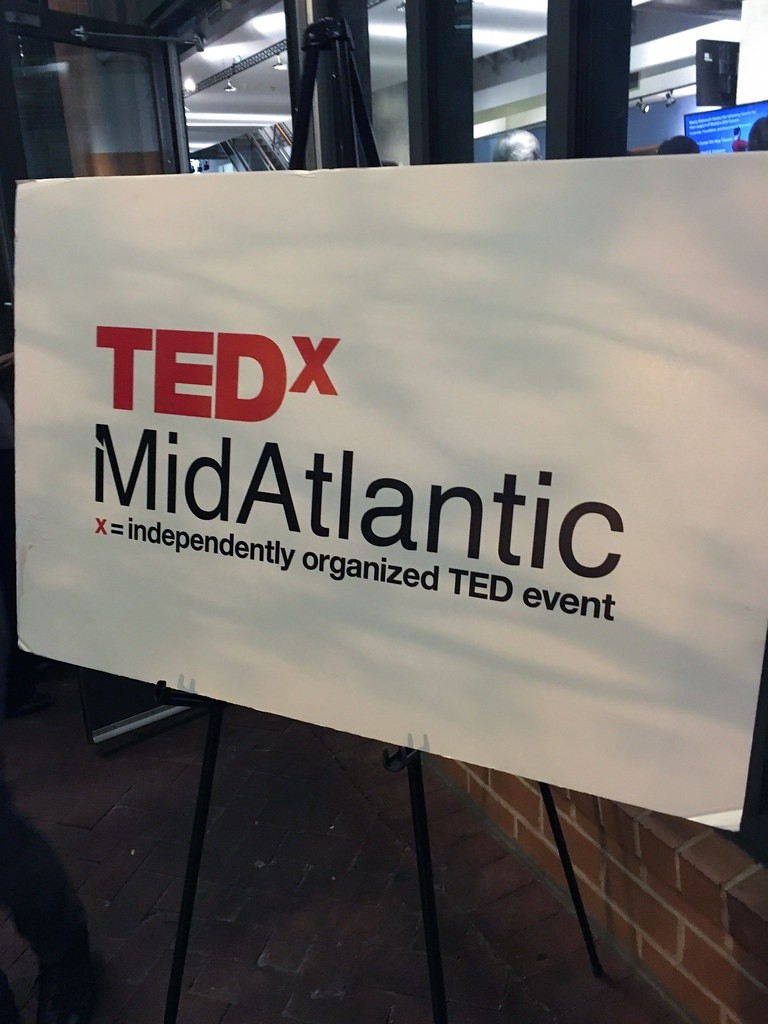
273,53 -> 288,71
665,90 -> 676,107
635,97 -> 650,114
225,77 -> 238,92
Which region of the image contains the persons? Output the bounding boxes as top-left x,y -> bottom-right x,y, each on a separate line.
732,127 -> 747,152
747,115 -> 768,152
492,128 -> 541,162
656,134 -> 701,156
